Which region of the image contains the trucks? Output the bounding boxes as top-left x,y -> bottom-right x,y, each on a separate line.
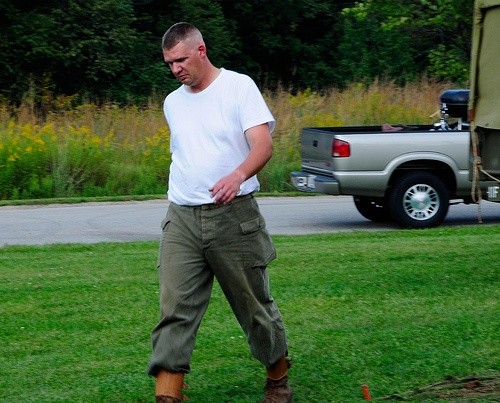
469,0 -> 500,205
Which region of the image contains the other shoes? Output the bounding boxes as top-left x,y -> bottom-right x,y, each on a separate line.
262,374 -> 292,403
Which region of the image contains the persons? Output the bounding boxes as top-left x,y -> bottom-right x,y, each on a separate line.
145,23 -> 295,403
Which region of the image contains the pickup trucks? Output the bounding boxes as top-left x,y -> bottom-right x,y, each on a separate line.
290,86 -> 481,226
